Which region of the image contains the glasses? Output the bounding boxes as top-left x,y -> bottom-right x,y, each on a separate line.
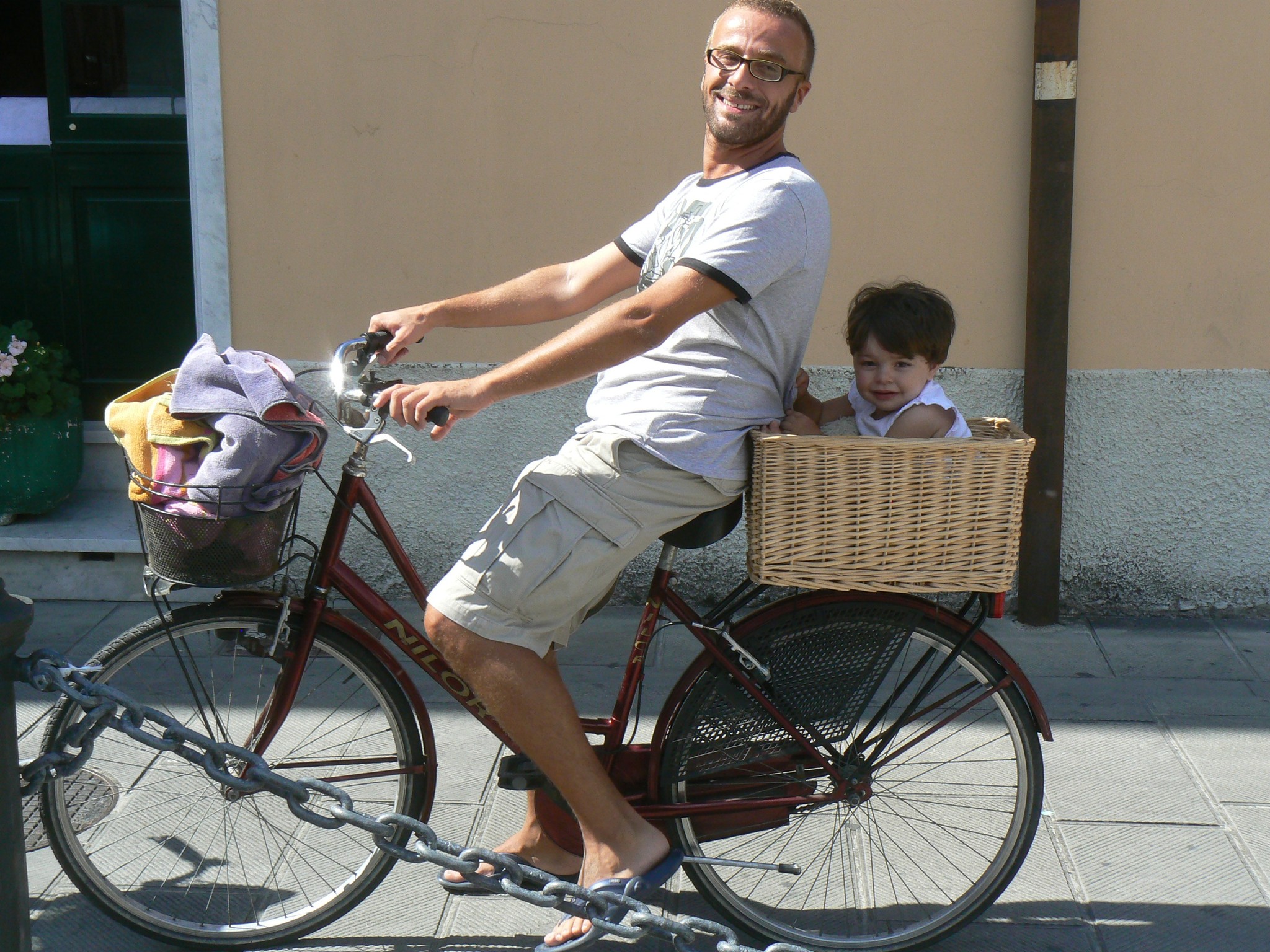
706,48 -> 806,82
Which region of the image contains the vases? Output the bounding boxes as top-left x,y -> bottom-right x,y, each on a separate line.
0,409 -> 84,524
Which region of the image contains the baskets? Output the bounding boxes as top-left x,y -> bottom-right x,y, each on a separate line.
122,447 -> 308,588
745,416 -> 1036,593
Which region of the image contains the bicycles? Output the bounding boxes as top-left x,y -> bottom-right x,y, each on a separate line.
28,325 -> 1058,952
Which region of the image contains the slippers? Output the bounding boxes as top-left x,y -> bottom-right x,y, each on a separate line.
534,848 -> 684,952
437,851 -> 581,894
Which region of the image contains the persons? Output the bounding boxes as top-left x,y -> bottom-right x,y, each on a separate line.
762,276 -> 982,475
368,0 -> 832,952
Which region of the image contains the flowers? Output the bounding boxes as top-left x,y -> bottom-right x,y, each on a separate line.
0,317 -> 85,419
